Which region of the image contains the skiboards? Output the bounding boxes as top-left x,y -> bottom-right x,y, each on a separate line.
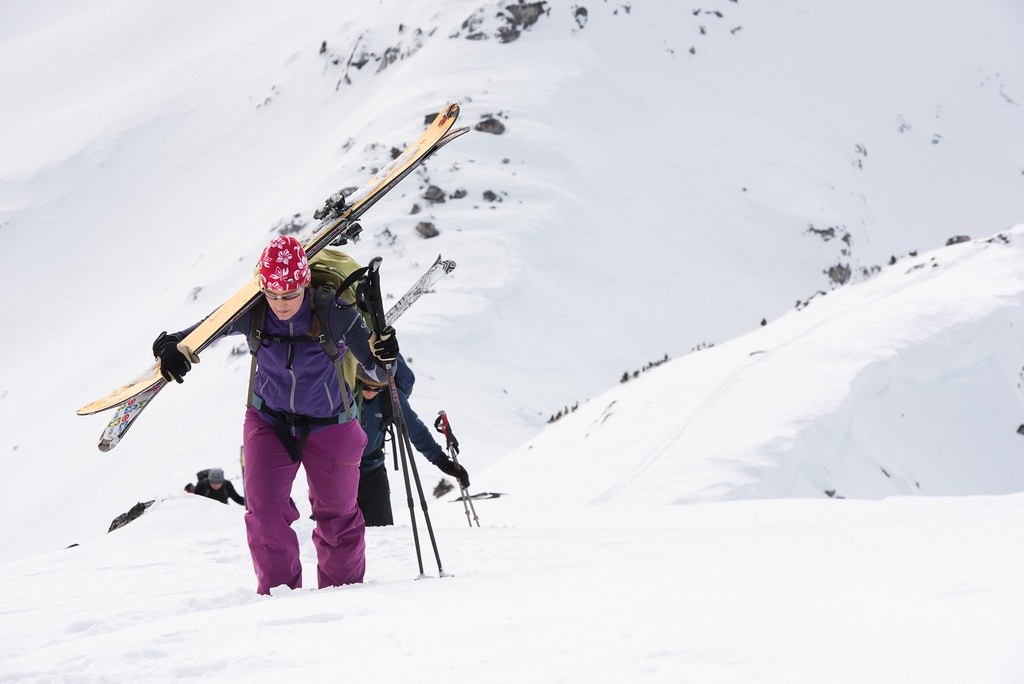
383,255 -> 456,326
77,100 -> 471,450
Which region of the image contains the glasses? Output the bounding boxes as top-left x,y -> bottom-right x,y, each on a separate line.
362,385 -> 384,393
261,288 -> 303,300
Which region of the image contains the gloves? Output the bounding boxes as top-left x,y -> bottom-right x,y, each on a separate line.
455,465 -> 469,490
152,332 -> 200,384
368,325 -> 399,370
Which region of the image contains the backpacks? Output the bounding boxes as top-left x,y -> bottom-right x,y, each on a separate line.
197,469 -> 228,499
247,249 -> 363,391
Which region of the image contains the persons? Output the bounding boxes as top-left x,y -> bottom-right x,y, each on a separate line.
193,467 -> 246,506
151,236 -> 402,598
353,353 -> 470,530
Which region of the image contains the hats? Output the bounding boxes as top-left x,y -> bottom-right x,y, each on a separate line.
209,468 -> 225,483
356,360 -> 397,386
259,236 -> 312,291
